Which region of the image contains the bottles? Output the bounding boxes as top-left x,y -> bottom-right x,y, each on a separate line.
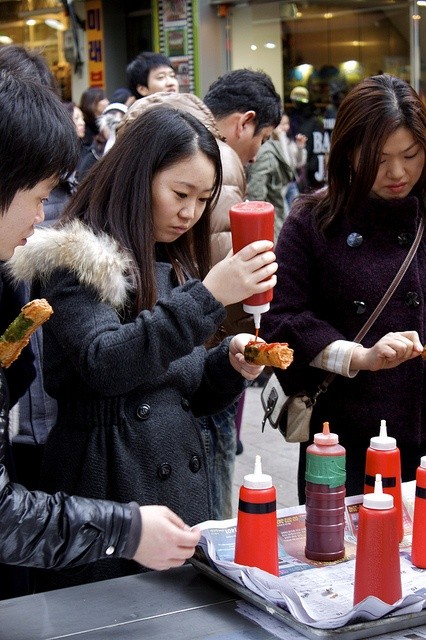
304,421 -> 347,559
229,201 -> 276,330
353,473 -> 402,605
234,455 -> 279,577
362,418 -> 405,540
410,454 -> 426,570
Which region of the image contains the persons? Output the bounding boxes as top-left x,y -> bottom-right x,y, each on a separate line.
60,99 -> 86,139
0,48 -> 77,446
78,87 -> 110,138
114,69 -> 283,350
78,103 -> 128,182
242,132 -> 292,224
276,112 -> 307,208
259,75 -> 425,503
126,53 -> 179,98
3,105 -> 279,529
0,66 -> 203,571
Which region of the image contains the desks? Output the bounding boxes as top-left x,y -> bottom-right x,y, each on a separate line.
1,565 -> 426,637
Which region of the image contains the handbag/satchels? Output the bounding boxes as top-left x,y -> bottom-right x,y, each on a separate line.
260,371 -> 312,443
